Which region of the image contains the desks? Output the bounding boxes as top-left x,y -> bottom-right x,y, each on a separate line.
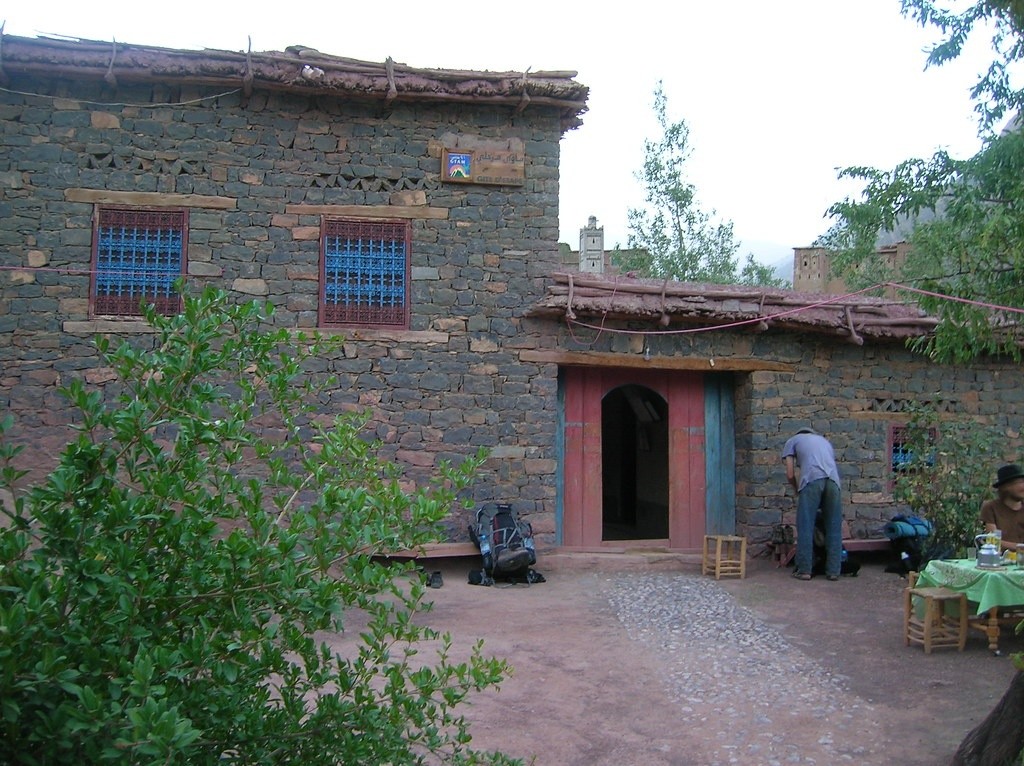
912,558 -> 1024,655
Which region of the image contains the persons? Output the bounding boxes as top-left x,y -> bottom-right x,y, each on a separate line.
781,430 -> 842,580
980,465 -> 1024,627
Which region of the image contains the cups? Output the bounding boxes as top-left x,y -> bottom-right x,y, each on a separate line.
980,529 -> 1001,555
1016,544 -> 1024,568
968,548 -> 976,561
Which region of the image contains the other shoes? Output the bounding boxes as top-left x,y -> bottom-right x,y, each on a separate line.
497,549 -> 529,567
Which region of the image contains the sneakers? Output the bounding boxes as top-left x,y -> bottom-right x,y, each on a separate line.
419,569 -> 431,585
771,526 -> 783,545
431,571 -> 443,588
784,528 -> 794,544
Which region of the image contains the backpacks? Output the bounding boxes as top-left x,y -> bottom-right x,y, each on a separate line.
473,503 -> 536,576
885,515 -> 932,575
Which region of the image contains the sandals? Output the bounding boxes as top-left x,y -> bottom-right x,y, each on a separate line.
792,571 -> 812,580
826,575 -> 839,581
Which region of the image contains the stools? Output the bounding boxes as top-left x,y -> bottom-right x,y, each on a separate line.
701,535 -> 747,580
905,587 -> 967,654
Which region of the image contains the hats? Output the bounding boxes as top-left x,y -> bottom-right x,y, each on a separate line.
794,427 -> 817,434
992,464 -> 1024,487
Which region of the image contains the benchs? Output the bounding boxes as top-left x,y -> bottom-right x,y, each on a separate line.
766,537 -> 891,568
349,541 -> 482,558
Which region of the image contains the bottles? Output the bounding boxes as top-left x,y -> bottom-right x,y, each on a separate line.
901,551 -> 909,560
480,535 -> 490,555
841,550 -> 847,562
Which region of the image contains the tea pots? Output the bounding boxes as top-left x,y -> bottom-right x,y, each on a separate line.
973,533 -> 1010,568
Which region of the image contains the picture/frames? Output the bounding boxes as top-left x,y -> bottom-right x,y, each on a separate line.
441,147 -> 475,183
